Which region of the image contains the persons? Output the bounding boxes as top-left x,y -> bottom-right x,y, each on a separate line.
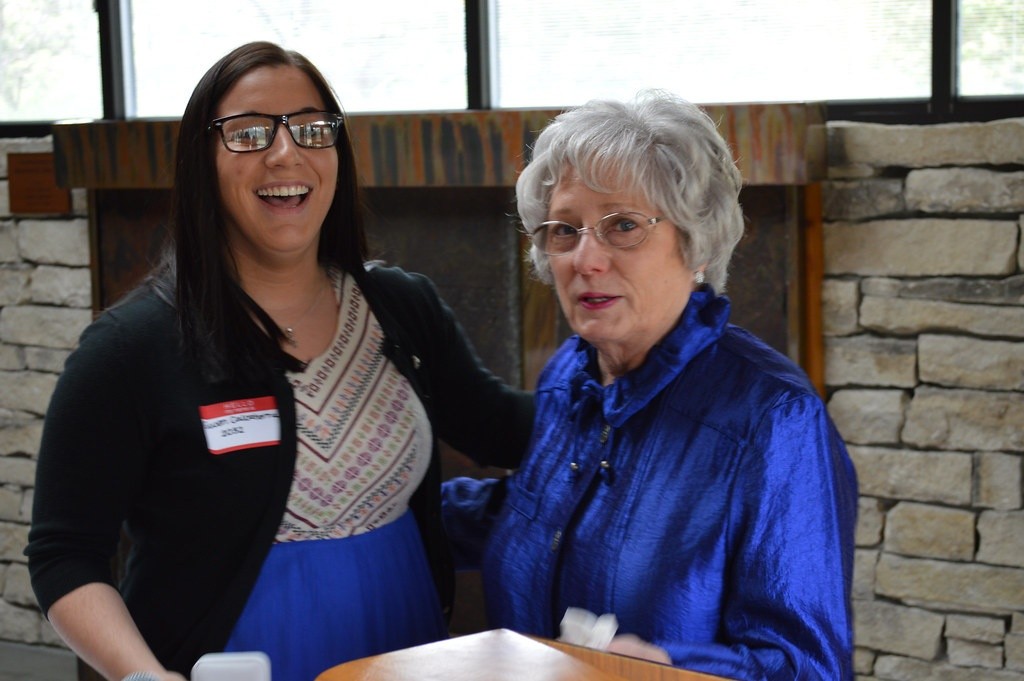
440,92 -> 860,681
23,42 -> 537,681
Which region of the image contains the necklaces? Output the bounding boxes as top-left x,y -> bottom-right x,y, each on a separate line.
281,278 -> 328,340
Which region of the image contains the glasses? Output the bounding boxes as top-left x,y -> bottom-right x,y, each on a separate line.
529,211 -> 666,257
207,109 -> 345,154
